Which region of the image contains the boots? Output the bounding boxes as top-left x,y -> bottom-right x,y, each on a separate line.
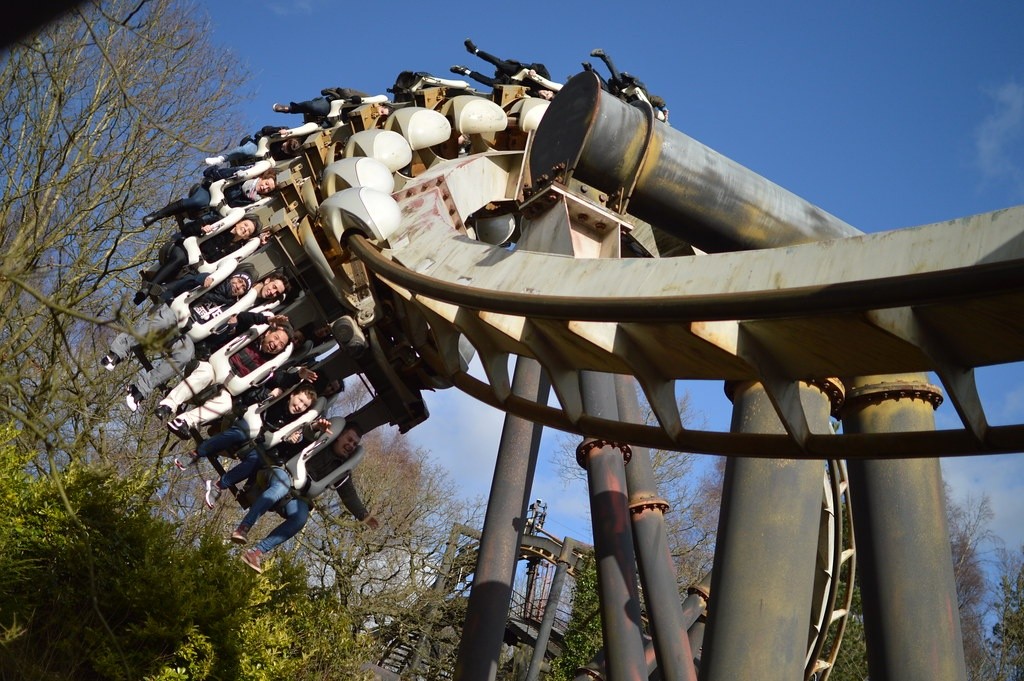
143,198 -> 184,228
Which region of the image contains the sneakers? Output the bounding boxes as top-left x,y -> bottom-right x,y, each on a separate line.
241,547 -> 263,573
100,350 -> 122,371
204,475 -> 221,509
174,448 -> 198,471
231,524 -> 251,544
166,418 -> 191,440
154,405 -> 172,421
125,383 -> 144,412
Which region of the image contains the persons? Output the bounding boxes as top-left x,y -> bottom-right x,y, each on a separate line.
99,39 -> 671,572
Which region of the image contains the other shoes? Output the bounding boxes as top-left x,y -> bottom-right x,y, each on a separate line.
463,38 -> 477,55
582,61 -> 592,71
272,103 -> 290,114
206,155 -> 225,166
240,135 -> 251,147
450,65 -> 468,76
590,49 -> 603,57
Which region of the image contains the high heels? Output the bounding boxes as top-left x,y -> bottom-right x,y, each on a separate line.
142,281 -> 166,297
133,287 -> 150,306
